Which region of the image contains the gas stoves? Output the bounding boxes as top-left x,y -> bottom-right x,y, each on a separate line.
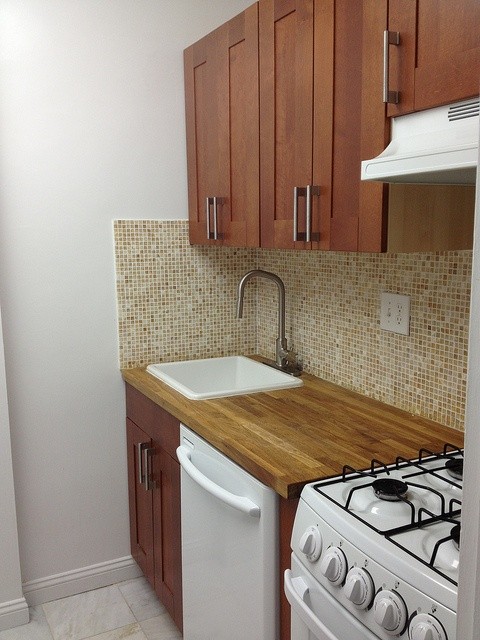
310,444 -> 464,586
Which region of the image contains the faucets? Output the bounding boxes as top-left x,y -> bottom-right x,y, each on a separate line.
235,270 -> 298,367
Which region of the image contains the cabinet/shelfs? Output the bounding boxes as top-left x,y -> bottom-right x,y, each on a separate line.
383,0 -> 479,116
182,1 -> 261,246
255,0 -> 387,251
124,382 -> 183,632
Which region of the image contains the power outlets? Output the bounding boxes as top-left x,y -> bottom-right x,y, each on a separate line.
379,293 -> 410,335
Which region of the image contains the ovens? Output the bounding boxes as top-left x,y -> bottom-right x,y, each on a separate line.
283,552 -> 382,640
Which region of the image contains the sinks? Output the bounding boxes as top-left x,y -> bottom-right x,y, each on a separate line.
146,356 -> 303,400
